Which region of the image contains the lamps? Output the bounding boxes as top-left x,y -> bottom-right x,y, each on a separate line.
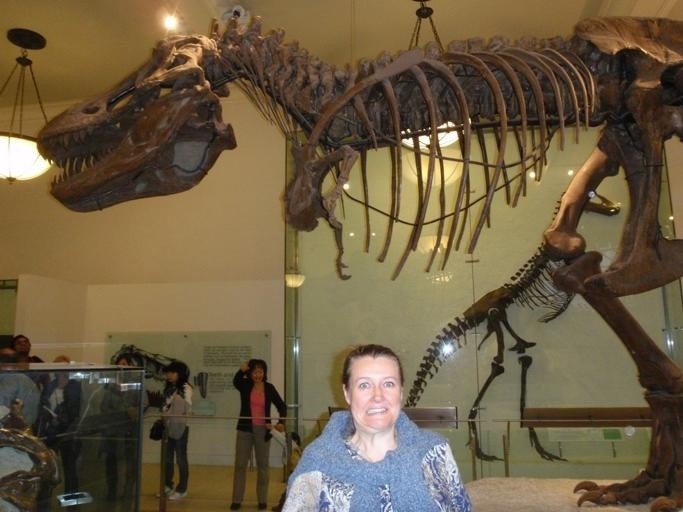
400,0 -> 471,151
0,28 -> 54,185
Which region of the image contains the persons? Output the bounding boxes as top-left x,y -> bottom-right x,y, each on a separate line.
279,343 -> 473,512
228,358 -> 288,511
0,332 -> 149,512
152,361 -> 194,500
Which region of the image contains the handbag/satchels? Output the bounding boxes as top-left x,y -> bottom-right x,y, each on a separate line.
44,403 -> 70,436
149,419 -> 164,441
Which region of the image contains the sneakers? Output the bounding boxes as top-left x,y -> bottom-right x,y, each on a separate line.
231,503 -> 241,510
168,491 -> 188,500
259,503 -> 266,510
156,487 -> 173,498
